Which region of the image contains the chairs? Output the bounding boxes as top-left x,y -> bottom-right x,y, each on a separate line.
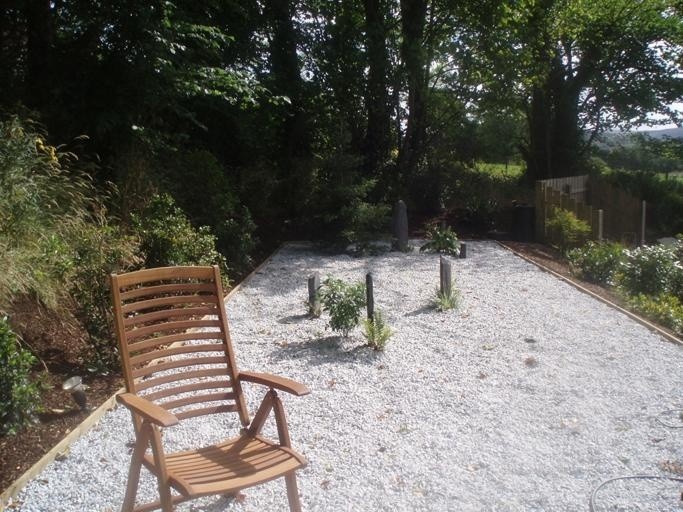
110,264 -> 313,512
